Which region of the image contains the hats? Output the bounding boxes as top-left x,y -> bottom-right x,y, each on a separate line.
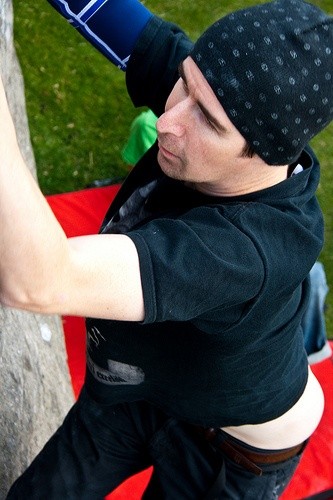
189,0 -> 333,166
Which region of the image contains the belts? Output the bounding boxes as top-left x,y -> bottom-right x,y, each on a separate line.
208,425 -> 303,476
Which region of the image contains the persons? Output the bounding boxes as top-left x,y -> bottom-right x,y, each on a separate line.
124,109 -> 328,354
0,0 -> 333,500
